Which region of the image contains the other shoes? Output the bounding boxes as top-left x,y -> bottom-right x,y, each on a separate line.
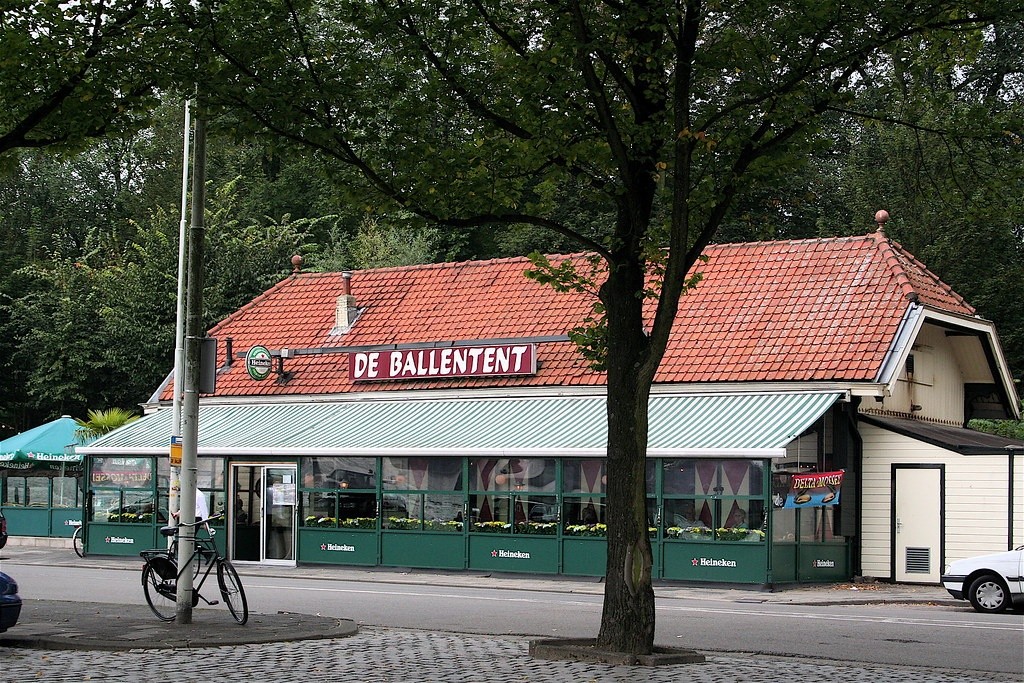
204,552 -> 214,565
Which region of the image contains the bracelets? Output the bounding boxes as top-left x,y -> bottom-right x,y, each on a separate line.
583,521 -> 586,522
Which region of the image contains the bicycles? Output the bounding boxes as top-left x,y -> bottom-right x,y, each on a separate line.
139,512 -> 248,625
72,523 -> 84,558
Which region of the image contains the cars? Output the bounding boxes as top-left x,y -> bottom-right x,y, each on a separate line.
0,572 -> 23,633
940,545 -> 1024,614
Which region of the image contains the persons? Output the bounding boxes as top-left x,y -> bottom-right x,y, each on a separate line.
172,486 -> 214,564
577,507 -> 594,525
729,509 -> 749,529
677,507 -> 706,535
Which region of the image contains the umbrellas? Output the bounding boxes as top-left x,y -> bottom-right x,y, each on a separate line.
0,415 -> 154,507
